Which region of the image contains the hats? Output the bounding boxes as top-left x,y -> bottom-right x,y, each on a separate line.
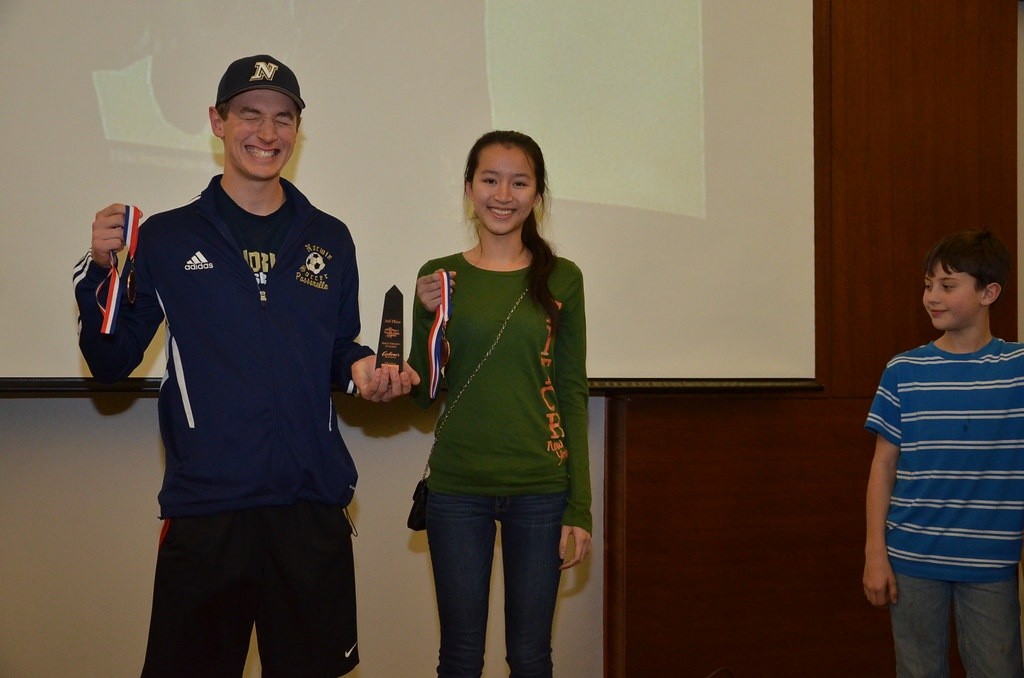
216,55 -> 305,110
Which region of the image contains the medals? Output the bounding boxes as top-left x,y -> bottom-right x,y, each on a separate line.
127,271 -> 137,303
441,338 -> 450,366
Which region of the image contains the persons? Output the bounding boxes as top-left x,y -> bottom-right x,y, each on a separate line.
74,54 -> 420,678
863,232 -> 1023,677
406,130 -> 592,676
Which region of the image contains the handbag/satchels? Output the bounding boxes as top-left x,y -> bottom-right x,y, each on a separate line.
407,478 -> 427,531
